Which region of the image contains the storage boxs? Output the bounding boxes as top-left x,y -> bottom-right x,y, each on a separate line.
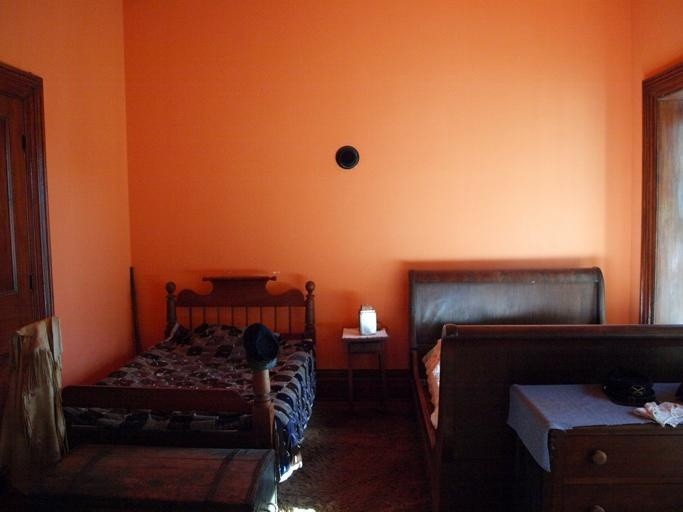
33,441 -> 278,512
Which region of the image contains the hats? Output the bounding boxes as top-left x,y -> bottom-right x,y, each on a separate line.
602,368 -> 660,407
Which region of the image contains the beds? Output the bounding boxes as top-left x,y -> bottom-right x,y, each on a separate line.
59,275 -> 319,512
407,266 -> 683,512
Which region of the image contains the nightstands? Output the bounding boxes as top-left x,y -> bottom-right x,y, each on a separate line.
341,328 -> 389,409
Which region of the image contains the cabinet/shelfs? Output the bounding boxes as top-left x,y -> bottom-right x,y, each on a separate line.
507,380 -> 683,512
